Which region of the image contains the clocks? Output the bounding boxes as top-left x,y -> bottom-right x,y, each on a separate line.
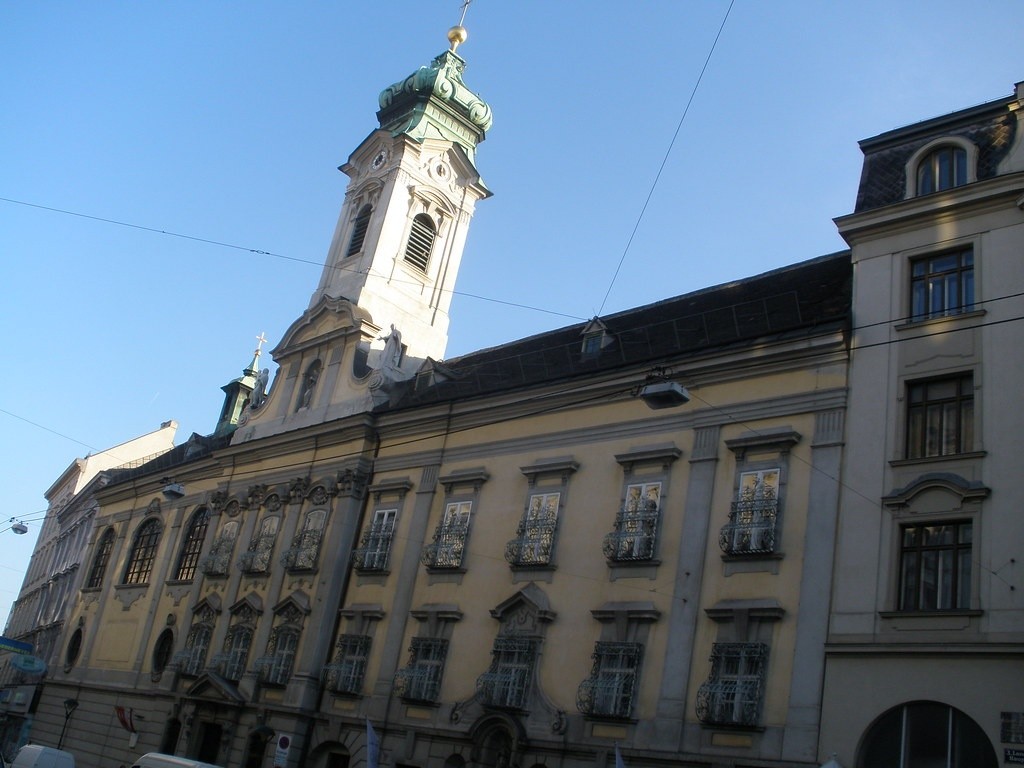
430,158 -> 452,183
372,151 -> 386,170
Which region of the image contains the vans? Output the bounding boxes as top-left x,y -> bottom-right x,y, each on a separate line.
10,745 -> 75,768
120,753 -> 219,768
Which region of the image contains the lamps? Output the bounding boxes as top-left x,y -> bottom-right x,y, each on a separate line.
162,484 -> 185,500
637,372 -> 690,410
11,524 -> 27,535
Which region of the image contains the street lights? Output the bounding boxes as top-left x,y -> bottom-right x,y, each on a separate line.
56,698 -> 80,749
